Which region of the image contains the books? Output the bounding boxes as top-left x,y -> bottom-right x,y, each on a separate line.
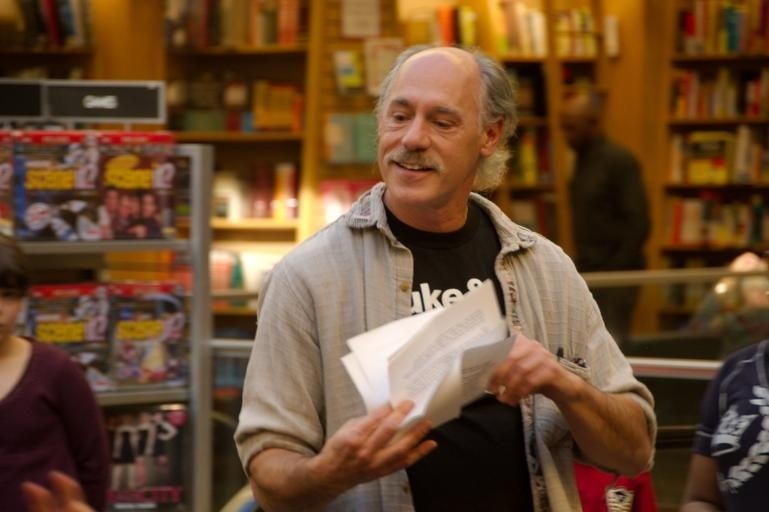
160,0 -> 768,319
1,128 -> 195,511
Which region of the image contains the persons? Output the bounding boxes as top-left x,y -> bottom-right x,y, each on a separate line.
553,88 -> 655,353
232,41 -> 658,512
675,331 -> 767,512
